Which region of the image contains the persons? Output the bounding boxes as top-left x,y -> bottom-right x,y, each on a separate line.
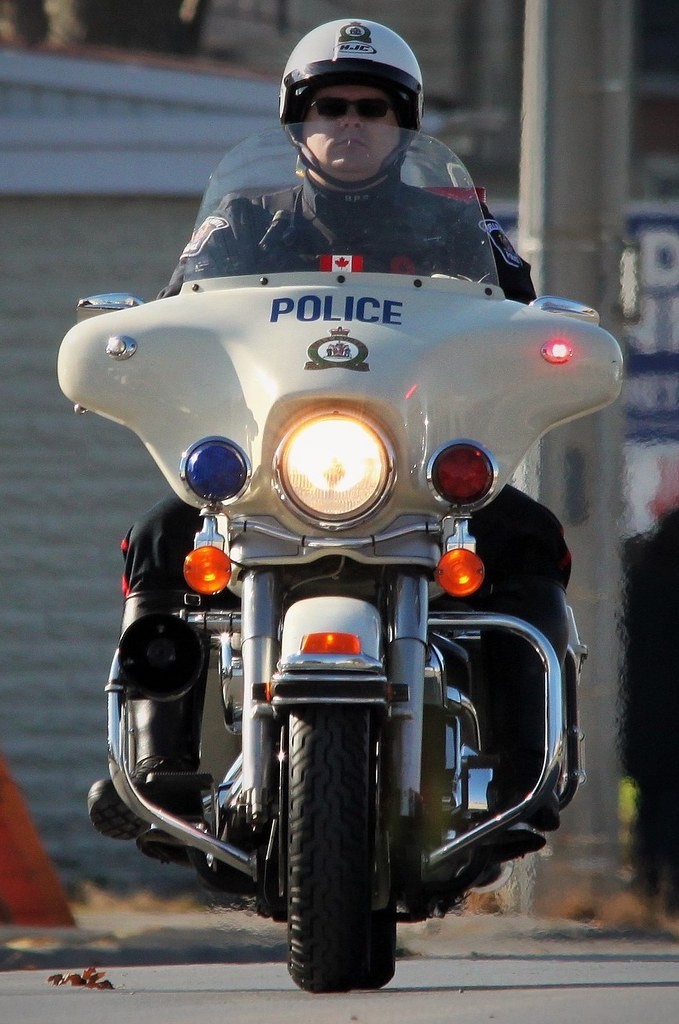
611,447 -> 679,943
86,17 -> 573,841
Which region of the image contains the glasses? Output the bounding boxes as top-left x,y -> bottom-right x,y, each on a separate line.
305,96 -> 398,119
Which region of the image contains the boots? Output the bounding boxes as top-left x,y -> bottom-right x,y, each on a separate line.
488,581 -> 568,829
87,593 -> 205,835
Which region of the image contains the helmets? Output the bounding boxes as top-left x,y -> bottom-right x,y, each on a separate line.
276,17 -> 424,128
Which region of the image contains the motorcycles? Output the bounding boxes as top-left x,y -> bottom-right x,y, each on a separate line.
56,110 -> 625,995
